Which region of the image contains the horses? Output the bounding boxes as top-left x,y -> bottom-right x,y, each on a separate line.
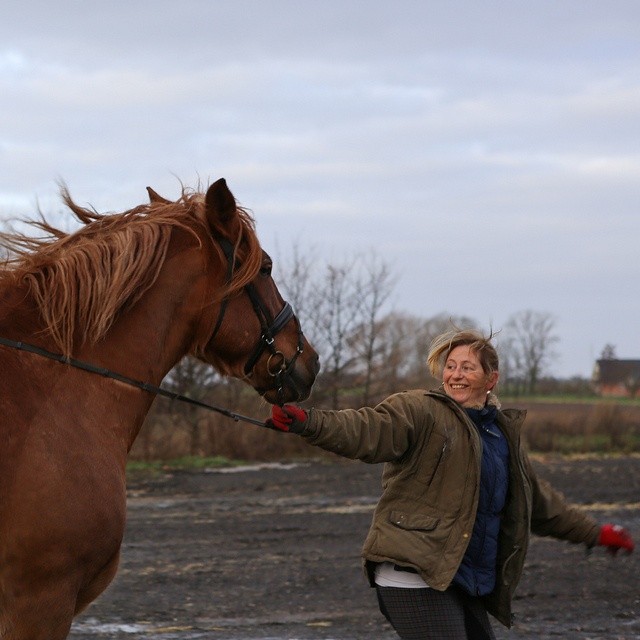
0,178 -> 321,640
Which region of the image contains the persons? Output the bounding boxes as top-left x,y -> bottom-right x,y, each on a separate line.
272,332 -> 635,636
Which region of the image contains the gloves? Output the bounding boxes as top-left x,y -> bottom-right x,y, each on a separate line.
600,523 -> 636,557
272,402 -> 307,432
